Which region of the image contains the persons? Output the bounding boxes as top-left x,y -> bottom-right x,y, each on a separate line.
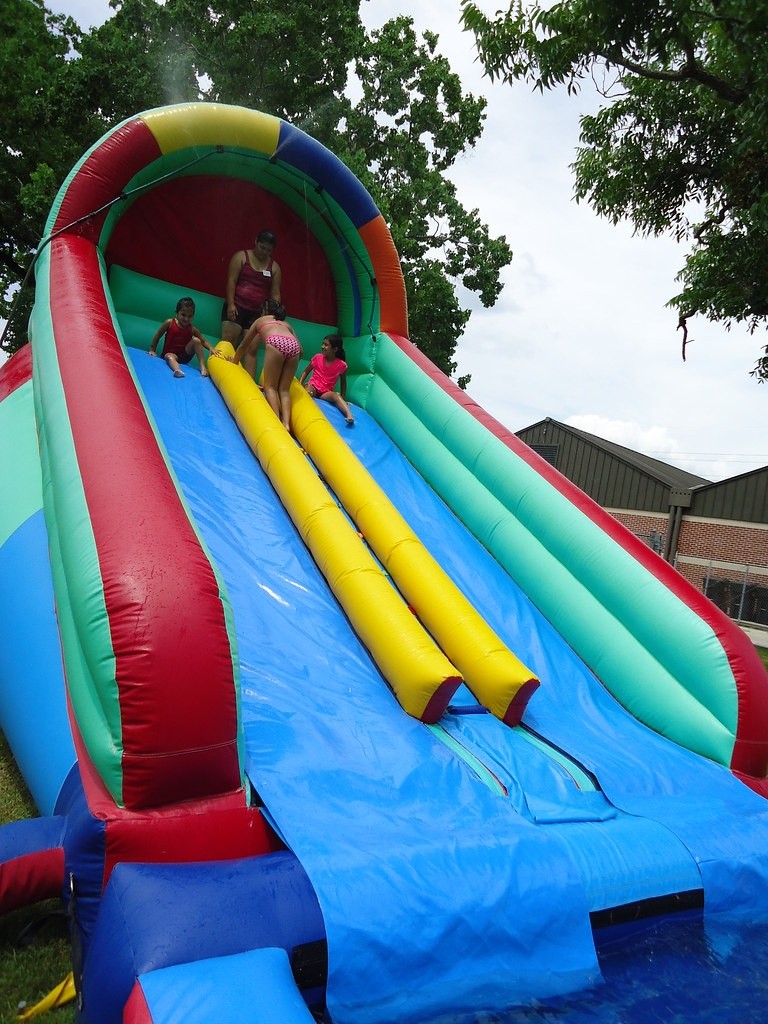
300,333 -> 355,423
224,298 -> 304,433
147,297 -> 223,378
221,228 -> 282,391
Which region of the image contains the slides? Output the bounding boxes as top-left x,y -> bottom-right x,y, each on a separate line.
29,233 -> 768,1024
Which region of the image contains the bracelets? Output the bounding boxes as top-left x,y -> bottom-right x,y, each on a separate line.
151,345 -> 155,349
